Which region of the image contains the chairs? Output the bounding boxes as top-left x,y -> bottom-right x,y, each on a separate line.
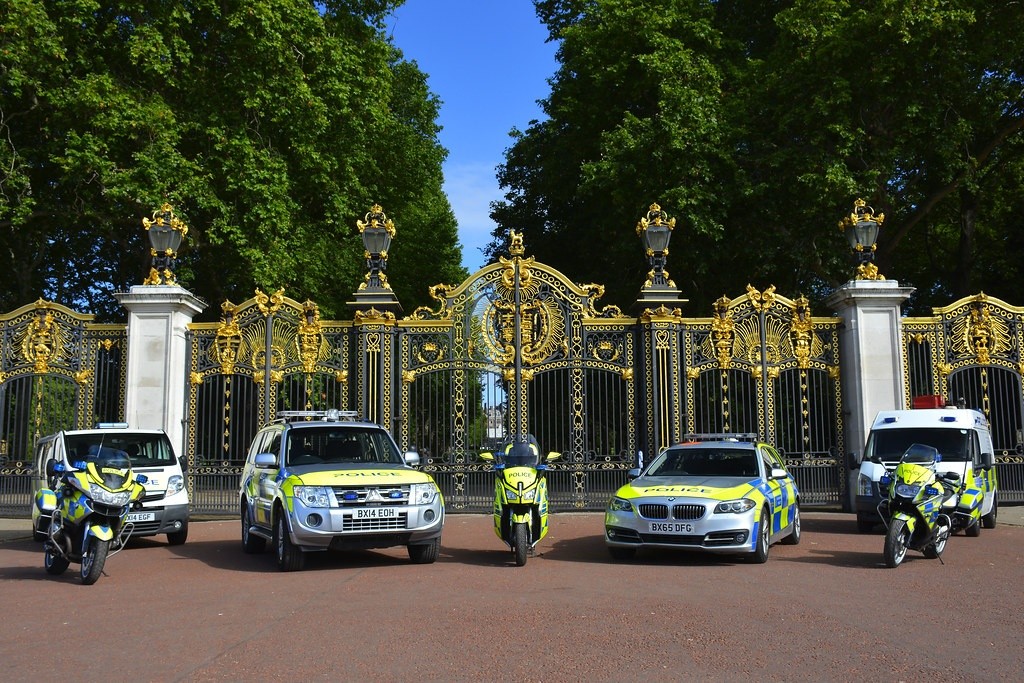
343,441 -> 366,460
120,444 -> 150,460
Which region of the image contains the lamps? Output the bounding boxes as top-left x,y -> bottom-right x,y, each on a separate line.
356,203 -> 396,292
837,197 -> 885,280
141,202 -> 189,286
636,202 -> 676,290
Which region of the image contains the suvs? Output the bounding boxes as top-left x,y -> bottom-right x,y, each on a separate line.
236,410 -> 445,566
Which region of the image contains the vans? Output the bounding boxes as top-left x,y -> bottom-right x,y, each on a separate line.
846,397 -> 999,538
32,424 -> 190,545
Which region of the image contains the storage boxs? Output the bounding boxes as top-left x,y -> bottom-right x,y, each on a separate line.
32,488 -> 57,532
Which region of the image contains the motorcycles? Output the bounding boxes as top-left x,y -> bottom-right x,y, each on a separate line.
33,445 -> 151,584
873,444 -> 959,568
478,434 -> 562,565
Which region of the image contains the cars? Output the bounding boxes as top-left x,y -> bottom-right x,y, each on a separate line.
604,433 -> 801,563
